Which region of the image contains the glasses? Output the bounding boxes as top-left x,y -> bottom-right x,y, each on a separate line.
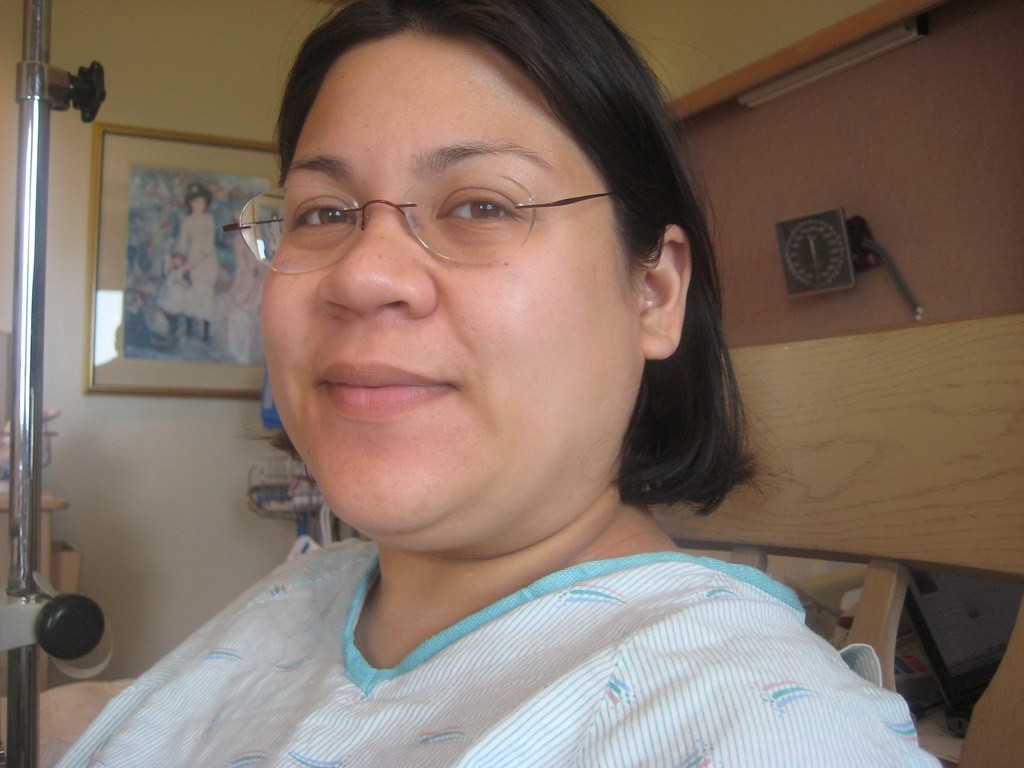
222,171 -> 613,267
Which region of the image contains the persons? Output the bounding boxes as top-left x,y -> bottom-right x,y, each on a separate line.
52,1 -> 949,768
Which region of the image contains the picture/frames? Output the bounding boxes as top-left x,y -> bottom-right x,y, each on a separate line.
80,122 -> 280,400
